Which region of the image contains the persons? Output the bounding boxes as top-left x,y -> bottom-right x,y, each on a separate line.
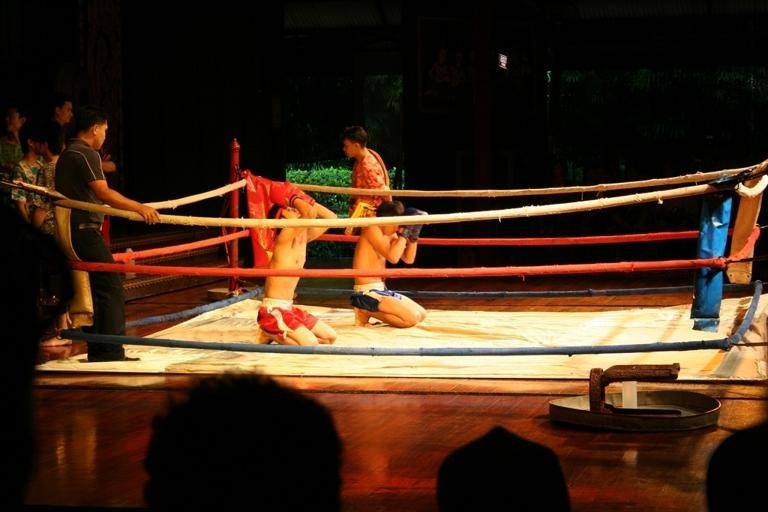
0,93 -> 118,349
256,181 -> 339,346
392,149 -> 768,283
54,105 -> 162,362
341,124 -> 393,292
436,421 -> 572,509
704,418 -> 768,511
350,200 -> 428,328
1,184 -> 75,512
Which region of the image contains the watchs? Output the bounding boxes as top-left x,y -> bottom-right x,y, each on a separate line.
140,367 -> 346,507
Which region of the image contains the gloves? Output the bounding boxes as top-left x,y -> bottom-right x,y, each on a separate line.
396,208 -> 428,243
268,180 -> 315,207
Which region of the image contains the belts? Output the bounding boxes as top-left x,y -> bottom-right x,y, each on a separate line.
70,221 -> 100,231
